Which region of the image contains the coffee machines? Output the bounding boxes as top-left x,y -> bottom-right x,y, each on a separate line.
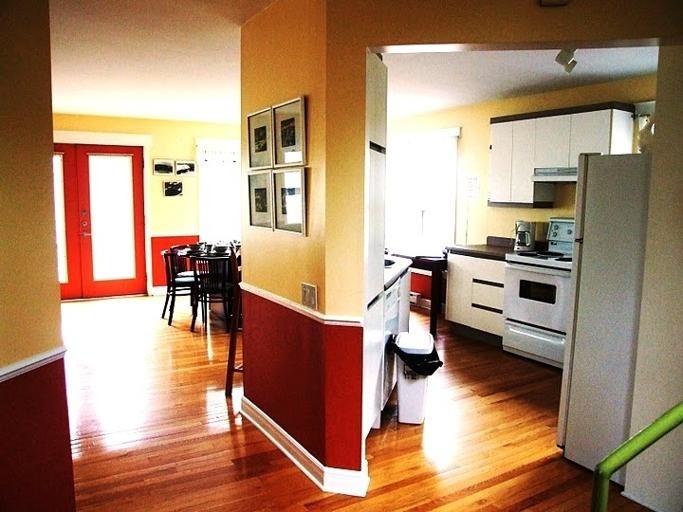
513,220 -> 536,251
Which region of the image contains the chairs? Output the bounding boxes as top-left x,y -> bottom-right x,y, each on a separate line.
160,241 -> 244,333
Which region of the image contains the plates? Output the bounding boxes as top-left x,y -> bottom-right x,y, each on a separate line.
187,251 -> 203,253
214,253 -> 229,256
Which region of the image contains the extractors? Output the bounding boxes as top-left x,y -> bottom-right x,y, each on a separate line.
531,168 -> 578,183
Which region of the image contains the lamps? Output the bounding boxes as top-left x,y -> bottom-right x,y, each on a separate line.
553,47 -> 578,74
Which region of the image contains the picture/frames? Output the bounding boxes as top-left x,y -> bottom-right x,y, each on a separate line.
247,95 -> 309,238
152,159 -> 195,198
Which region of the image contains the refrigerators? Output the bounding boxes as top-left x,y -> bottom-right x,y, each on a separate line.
556,152 -> 652,486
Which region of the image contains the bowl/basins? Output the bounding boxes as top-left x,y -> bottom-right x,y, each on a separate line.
189,244 -> 200,250
213,245 -> 228,252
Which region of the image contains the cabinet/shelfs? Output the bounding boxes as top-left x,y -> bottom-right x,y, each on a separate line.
445,252 -> 504,346
489,101 -> 635,208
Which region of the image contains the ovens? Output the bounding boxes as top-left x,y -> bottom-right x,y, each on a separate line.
501,260 -> 572,370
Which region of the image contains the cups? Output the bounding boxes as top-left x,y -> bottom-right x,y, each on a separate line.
205,244 -> 213,254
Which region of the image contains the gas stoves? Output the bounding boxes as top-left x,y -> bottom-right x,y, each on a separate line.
508,251 -> 573,264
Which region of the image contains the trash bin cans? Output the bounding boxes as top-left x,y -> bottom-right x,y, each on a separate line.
395,332 -> 435,424
